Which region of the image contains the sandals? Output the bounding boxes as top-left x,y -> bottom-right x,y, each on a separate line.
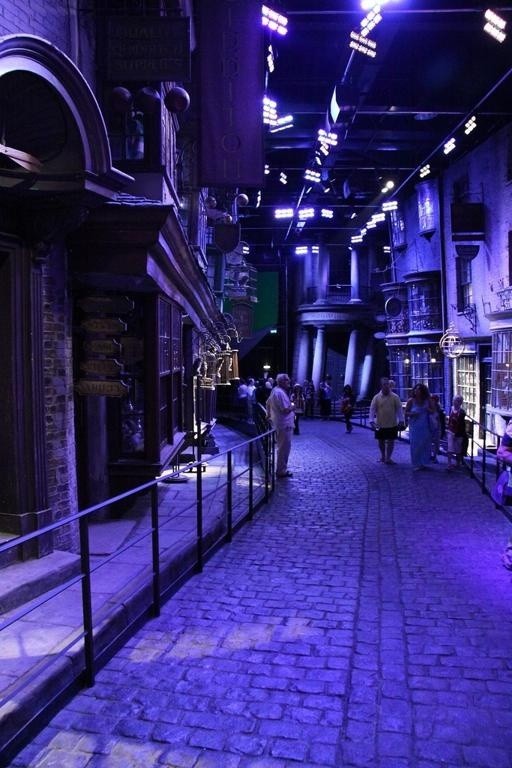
276,470 -> 293,478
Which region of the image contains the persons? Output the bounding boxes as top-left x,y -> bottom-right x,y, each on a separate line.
447,396 -> 468,471
237,378 -> 356,442
497,417 -> 512,570
404,384 -> 435,471
429,395 -> 445,463
265,373 -> 297,478
369,377 -> 404,465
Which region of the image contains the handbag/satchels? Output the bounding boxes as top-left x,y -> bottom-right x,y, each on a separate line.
491,471 -> 512,506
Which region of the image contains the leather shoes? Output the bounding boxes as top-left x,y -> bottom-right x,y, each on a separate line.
376,458 -> 397,465
